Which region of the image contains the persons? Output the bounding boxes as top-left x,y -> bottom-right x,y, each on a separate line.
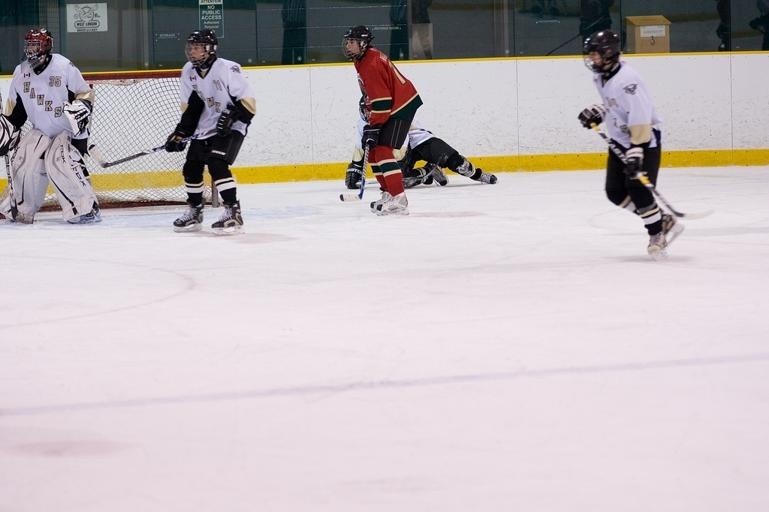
578,31 -> 674,254
342,26 -> 423,212
166,27 -> 255,228
345,95 -> 497,189
0,26 -> 101,224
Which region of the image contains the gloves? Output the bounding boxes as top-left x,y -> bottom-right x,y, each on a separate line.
578,105 -> 603,129
345,164 -> 362,189
622,146 -> 644,180
165,132 -> 187,152
216,107 -> 237,138
361,124 -> 382,150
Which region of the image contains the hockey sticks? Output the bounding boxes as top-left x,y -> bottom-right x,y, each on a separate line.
340,144 -> 370,202
89,128 -> 218,169
0,89 -> 34,224
590,121 -> 715,221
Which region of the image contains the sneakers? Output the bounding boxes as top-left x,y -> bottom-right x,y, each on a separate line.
477,172 -> 497,184
647,215 -> 674,250
212,201 -> 243,228
426,161 -> 447,186
370,192 -> 408,212
173,205 -> 203,227
67,208 -> 101,224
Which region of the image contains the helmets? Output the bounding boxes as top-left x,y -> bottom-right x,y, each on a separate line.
342,26 -> 375,59
24,28 -> 53,61
583,30 -> 619,73
185,28 -> 218,68
